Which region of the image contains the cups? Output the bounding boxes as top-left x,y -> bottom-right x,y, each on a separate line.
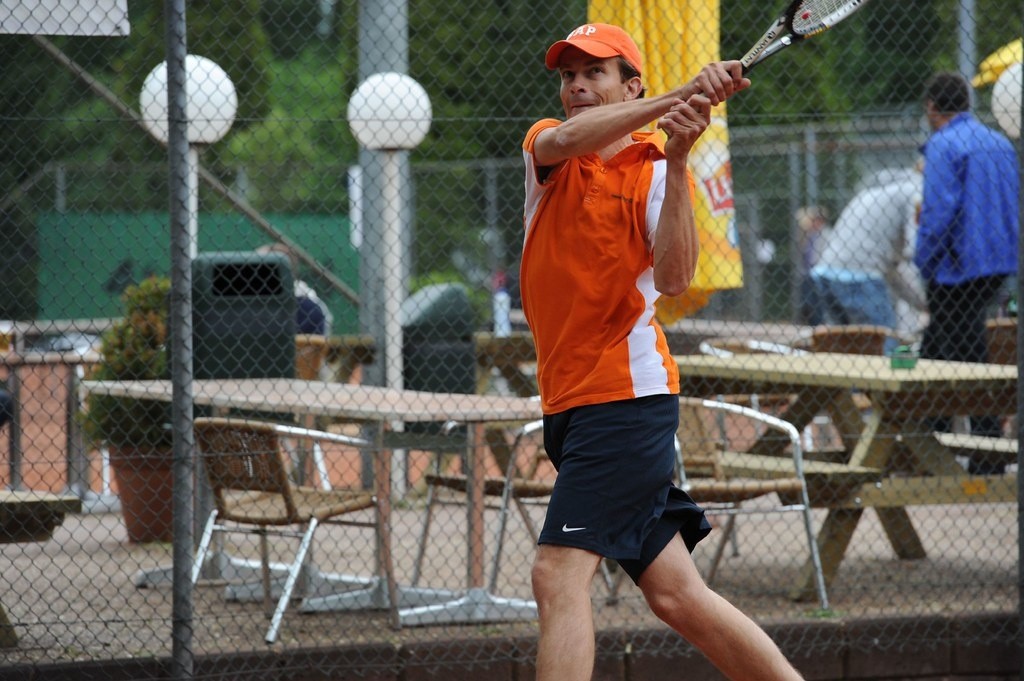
1,327 -> 12,354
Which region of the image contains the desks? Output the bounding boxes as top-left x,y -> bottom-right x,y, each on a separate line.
664,352 -> 1020,604
81,375 -> 544,628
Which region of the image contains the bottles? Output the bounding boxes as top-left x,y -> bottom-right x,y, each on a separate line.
492,287 -> 514,338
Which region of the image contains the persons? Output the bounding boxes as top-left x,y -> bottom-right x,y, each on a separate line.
518,23 -> 806,681
812,180 -> 928,357
256,241 -> 333,337
798,203 -> 833,322
915,76 -> 1020,474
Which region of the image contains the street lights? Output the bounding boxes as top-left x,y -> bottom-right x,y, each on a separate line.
346,72 -> 433,501
137,48 -> 240,573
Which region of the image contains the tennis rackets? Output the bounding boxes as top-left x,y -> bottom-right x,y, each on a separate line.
662,0 -> 872,139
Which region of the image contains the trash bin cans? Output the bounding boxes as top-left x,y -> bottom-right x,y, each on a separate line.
403,282 -> 477,440
192,250 -> 295,424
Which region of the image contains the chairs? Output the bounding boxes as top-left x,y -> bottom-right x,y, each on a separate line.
410,420 -> 617,600
187,416 -> 377,644
284,281 -> 909,508
671,396 -> 835,618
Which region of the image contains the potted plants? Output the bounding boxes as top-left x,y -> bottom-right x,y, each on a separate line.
83,275 -> 198,546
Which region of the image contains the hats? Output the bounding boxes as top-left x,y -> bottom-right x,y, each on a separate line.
546,22 -> 643,77
